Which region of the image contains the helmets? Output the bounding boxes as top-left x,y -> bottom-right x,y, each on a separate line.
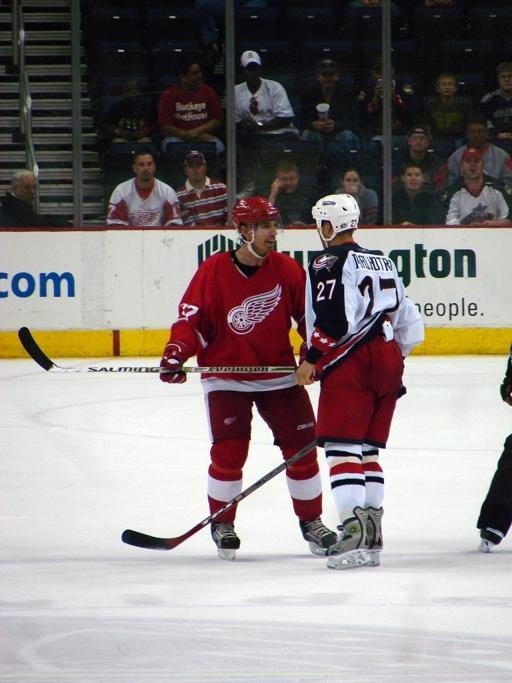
312,193 -> 362,241
232,197 -> 282,244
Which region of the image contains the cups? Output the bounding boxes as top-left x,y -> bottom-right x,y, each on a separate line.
316,102 -> 330,119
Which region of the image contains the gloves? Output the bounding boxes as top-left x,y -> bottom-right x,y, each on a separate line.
160,341 -> 192,385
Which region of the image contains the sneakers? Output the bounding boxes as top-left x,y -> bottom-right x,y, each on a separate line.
299,517 -> 339,549
365,506 -> 384,551
211,522 -> 241,550
326,506 -> 369,558
480,528 -> 505,546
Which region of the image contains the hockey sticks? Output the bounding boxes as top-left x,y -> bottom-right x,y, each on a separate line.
17,325 -> 314,374
121,438 -> 325,552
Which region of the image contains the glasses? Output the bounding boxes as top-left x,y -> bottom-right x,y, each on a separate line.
250,97 -> 259,115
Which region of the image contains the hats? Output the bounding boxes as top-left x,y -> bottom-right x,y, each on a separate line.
317,59 -> 338,75
461,148 -> 483,161
241,50 -> 263,68
184,149 -> 207,167
408,124 -> 430,139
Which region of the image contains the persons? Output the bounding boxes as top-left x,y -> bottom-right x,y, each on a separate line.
156,191 -> 336,559
473,338 -> 512,557
1,168 -> 71,227
293,193 -> 427,568
104,43 -> 512,227
